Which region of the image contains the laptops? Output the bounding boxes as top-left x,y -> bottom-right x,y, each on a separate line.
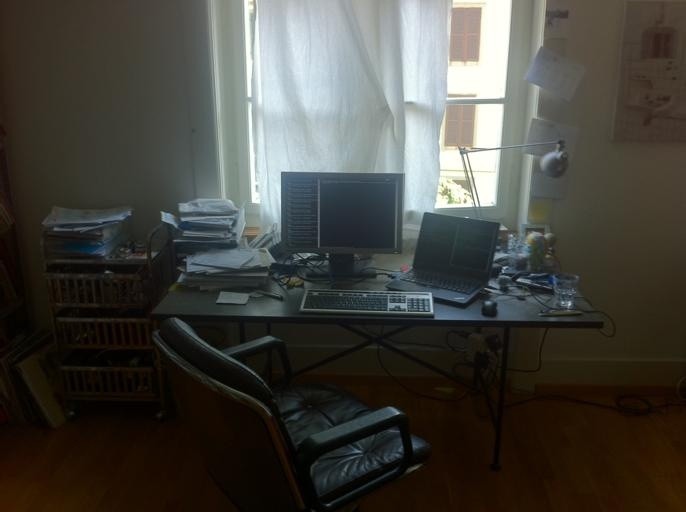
384,211 -> 500,307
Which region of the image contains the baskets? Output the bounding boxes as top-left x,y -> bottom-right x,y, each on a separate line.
47,251 -> 166,309
54,308 -> 161,352
56,352 -> 161,397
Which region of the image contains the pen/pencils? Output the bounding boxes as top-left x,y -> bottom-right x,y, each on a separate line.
539,310 -> 584,316
256,291 -> 284,301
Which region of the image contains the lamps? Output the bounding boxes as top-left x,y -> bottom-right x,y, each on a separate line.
457,139 -> 569,220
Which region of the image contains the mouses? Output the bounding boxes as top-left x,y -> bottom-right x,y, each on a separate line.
482,300 -> 497,317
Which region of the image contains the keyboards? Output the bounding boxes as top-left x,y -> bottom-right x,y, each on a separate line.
300,288 -> 434,318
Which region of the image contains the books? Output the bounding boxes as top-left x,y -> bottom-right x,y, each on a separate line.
40,205 -> 133,258
0,188 -> 77,429
159,199 -> 278,292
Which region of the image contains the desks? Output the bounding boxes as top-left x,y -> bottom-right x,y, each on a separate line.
150,255 -> 603,472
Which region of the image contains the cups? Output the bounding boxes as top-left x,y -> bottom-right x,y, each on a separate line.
553,273 -> 579,311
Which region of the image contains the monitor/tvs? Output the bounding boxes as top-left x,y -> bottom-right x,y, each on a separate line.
280,172 -> 405,278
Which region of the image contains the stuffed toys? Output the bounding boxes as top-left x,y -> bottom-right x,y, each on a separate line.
522,231 -> 551,274
543,231 -> 561,275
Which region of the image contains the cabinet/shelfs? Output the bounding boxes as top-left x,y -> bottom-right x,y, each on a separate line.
40,221 -> 175,423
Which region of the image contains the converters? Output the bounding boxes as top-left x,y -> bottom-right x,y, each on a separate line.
511,382 -> 536,396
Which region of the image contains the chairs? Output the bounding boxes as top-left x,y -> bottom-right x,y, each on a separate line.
150,318 -> 433,512
